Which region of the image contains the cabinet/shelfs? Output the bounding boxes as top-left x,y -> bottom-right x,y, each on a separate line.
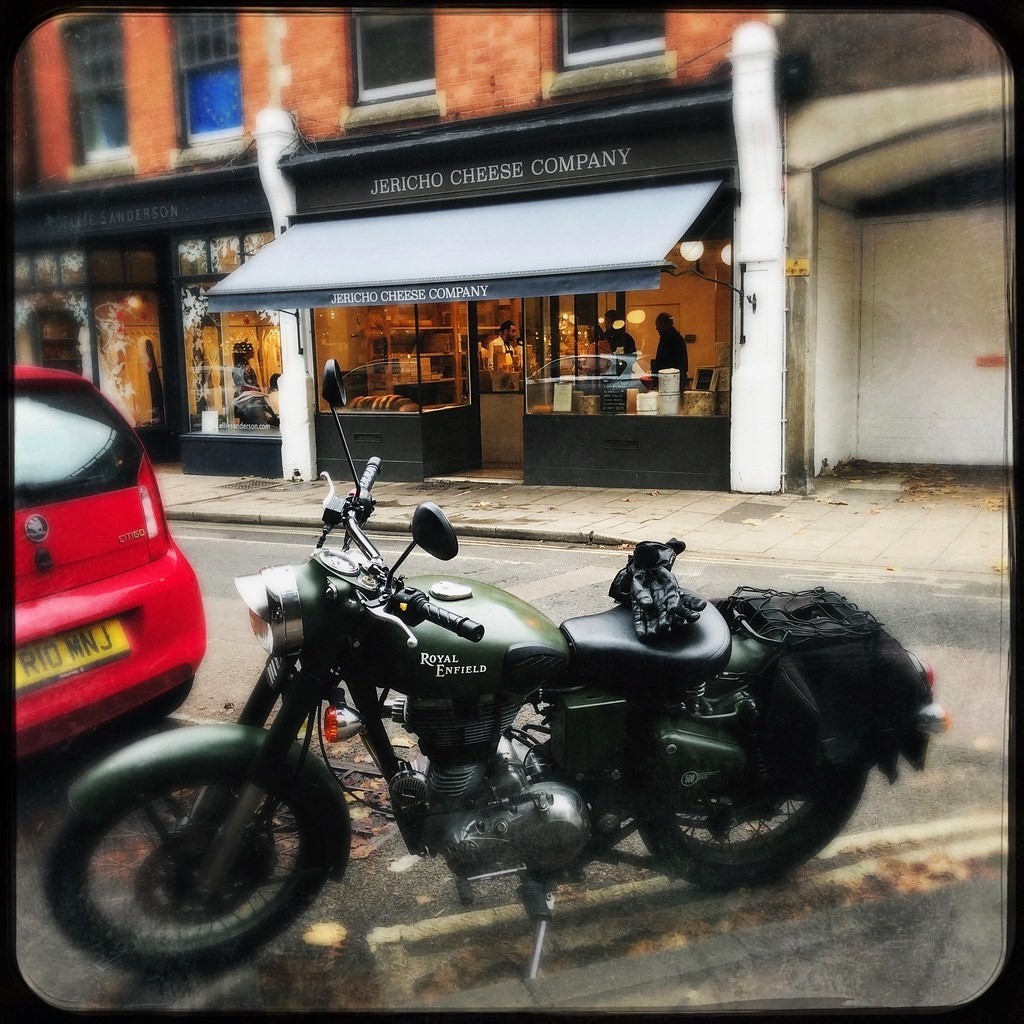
365,300 -> 525,406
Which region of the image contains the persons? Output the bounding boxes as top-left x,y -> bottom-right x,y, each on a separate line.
605,310 -> 637,374
488,321 -> 516,369
652,312 -> 688,389
577,325 -> 611,376
232,343 -> 281,427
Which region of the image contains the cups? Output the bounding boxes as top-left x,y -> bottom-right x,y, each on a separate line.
627,389 -> 639,413
572,391 -> 584,413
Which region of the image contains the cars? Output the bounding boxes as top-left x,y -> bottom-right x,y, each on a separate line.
11,364 -> 205,758
525,354 -> 657,415
343,360 -> 448,414
188,366 -> 262,425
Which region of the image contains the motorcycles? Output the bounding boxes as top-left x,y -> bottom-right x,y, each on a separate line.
44,360 -> 949,976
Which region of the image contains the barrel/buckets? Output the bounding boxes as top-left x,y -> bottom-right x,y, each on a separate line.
577,395 -> 600,414
638,392 -> 658,414
657,368 -> 681,416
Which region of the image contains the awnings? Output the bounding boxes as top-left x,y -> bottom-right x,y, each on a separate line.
203,175 -> 724,313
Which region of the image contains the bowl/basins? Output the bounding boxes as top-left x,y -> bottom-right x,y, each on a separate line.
641,376 -> 659,390
